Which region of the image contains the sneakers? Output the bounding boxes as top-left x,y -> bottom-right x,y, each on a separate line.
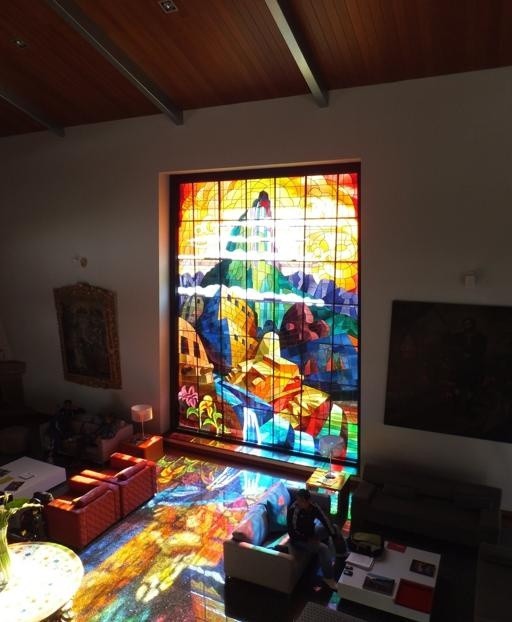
322,577 -> 338,590
336,552 -> 351,558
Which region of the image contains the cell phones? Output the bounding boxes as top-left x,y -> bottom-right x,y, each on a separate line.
345,571 -> 353,576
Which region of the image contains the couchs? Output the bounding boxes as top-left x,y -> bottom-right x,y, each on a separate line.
41,414 -> 134,466
350,459 -> 503,549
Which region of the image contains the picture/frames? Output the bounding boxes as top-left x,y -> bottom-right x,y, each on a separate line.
52,281 -> 122,390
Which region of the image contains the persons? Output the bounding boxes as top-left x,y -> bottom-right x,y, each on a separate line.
286,489 -> 343,591
49,398 -> 118,466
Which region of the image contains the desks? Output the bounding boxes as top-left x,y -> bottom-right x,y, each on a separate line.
0,540 -> 85,622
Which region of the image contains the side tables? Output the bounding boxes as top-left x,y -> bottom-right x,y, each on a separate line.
122,433 -> 164,463
305,466 -> 350,530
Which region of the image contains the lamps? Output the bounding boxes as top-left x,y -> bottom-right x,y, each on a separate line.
130,404 -> 152,439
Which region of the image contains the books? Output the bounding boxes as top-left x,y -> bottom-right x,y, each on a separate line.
344,552 -> 376,570
362,573 -> 394,596
409,559 -> 435,577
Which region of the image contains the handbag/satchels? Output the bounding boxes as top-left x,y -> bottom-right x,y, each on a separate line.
268,545 -> 289,554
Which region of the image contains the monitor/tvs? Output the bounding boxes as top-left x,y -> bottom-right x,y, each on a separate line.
383,299 -> 512,444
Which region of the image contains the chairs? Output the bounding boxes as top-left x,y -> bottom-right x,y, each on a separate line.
222,498 -> 321,595
45,451 -> 158,553
